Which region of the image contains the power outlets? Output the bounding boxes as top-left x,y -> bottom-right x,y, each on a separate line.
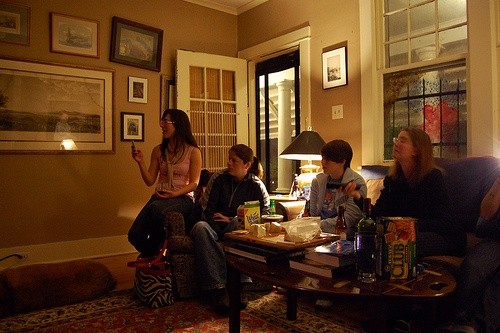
15,252 -> 32,262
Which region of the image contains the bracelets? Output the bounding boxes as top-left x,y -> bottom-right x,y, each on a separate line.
229,217 -> 233,221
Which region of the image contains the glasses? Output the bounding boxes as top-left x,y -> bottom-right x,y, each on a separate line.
393,137 -> 414,148
159,120 -> 175,126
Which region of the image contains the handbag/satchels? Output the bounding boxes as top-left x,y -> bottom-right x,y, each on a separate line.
125,238 -> 175,307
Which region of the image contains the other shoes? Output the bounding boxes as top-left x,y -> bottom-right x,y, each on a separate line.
315,299 -> 333,307
214,296 -> 249,313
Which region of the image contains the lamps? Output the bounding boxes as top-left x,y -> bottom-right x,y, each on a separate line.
279,131 -> 327,185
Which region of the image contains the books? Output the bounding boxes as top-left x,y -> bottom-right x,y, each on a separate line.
224,244 -> 288,264
289,250 -> 357,278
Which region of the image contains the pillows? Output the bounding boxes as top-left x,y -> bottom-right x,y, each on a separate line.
363,178 -> 384,206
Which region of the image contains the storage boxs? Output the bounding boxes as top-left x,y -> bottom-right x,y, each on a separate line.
380,216 -> 416,280
237,200 -> 261,229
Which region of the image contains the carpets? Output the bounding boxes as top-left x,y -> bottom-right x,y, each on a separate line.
0,278 -> 356,333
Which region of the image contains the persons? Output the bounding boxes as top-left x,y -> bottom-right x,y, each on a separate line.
294,139 -> 367,311
191,144 -> 271,313
128,109 -> 201,256
341,127 -> 452,258
446,173 -> 500,333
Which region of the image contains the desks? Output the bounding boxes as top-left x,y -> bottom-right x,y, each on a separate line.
225,251 -> 457,333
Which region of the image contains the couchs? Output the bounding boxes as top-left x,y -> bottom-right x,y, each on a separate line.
165,168 -> 271,300
274,156 -> 500,333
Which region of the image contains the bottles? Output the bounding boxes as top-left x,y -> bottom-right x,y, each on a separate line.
375,216 -> 390,281
358,198 -> 376,233
292,181 -> 300,194
335,205 -> 347,241
269,199 -> 276,215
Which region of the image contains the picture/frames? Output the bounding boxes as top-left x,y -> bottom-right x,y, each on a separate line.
0,0 -> 32,47
109,15 -> 163,72
50,12 -> 100,58
161,75 -> 176,128
321,46 -> 348,90
0,54 -> 116,155
128,76 -> 148,104
121,112 -> 145,142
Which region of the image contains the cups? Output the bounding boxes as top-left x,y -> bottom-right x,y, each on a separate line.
356,234 -> 377,283
